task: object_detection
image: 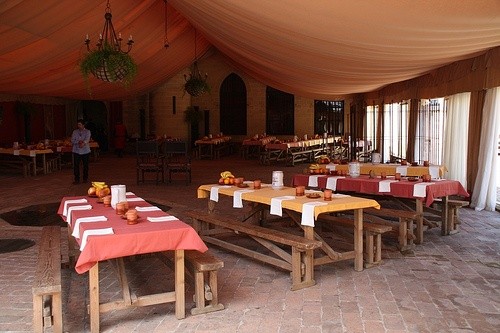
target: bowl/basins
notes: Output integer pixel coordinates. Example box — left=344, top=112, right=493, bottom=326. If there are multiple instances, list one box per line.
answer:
left=411, top=161, right=418, bottom=166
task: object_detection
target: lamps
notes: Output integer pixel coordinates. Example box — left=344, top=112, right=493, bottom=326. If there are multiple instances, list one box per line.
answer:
left=182, top=32, right=211, bottom=98
left=80, top=0, right=137, bottom=97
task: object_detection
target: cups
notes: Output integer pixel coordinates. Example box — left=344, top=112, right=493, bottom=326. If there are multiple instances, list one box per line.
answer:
left=395, top=173, right=401, bottom=181
left=421, top=174, right=431, bottom=182
left=381, top=171, right=386, bottom=180
left=401, top=158, right=407, bottom=164
left=254, top=180, right=261, bottom=189
left=296, top=185, right=305, bottom=196
left=324, top=190, right=332, bottom=200
left=369, top=170, right=377, bottom=178
left=103, top=195, right=111, bottom=207
left=126, top=209, right=138, bottom=224
left=116, top=201, right=129, bottom=215
left=424, top=160, right=429, bottom=166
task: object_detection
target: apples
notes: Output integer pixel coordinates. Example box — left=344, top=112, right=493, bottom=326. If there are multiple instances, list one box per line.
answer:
left=87, top=187, right=110, bottom=197
left=310, top=169, right=319, bottom=173
left=219, top=178, right=234, bottom=184
left=320, top=159, right=328, bottom=164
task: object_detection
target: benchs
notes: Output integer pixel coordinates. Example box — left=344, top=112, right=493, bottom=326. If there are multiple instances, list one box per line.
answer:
left=56, top=192, right=208, bottom=333
left=186, top=193, right=469, bottom=333
left=31, top=223, right=63, bottom=333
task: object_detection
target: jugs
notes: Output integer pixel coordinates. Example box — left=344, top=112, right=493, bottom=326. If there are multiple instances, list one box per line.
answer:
left=372, top=153, right=380, bottom=165
left=272, top=171, right=284, bottom=189
left=349, top=162, right=361, bottom=178
left=110, top=184, right=126, bottom=209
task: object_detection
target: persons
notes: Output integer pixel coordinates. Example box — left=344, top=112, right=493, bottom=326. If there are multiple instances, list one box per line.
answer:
left=72, top=120, right=91, bottom=185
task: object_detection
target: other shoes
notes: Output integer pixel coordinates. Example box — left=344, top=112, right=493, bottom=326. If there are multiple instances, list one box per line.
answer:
left=72, top=181, right=80, bottom=184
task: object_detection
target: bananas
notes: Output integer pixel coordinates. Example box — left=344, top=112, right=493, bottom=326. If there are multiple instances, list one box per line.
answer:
left=320, top=156, right=327, bottom=159
left=221, top=171, right=235, bottom=178
left=91, top=181, right=108, bottom=189
left=310, top=164, right=319, bottom=169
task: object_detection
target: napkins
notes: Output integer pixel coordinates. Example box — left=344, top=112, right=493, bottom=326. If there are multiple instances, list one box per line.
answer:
left=62, top=163, right=438, bottom=257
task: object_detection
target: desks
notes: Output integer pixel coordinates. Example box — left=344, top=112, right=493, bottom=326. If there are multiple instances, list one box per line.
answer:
left=197, top=181, right=381, bottom=281
left=290, top=174, right=470, bottom=244
left=304, top=163, right=429, bottom=177
left=194, top=136, right=342, bottom=166
left=0, top=143, right=98, bottom=176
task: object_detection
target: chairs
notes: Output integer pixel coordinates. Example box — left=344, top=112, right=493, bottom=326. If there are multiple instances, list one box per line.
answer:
left=136, top=141, right=192, bottom=186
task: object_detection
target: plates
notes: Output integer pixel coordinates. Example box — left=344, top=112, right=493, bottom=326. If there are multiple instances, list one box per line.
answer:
left=307, top=193, right=321, bottom=199
left=408, top=177, right=419, bottom=181
